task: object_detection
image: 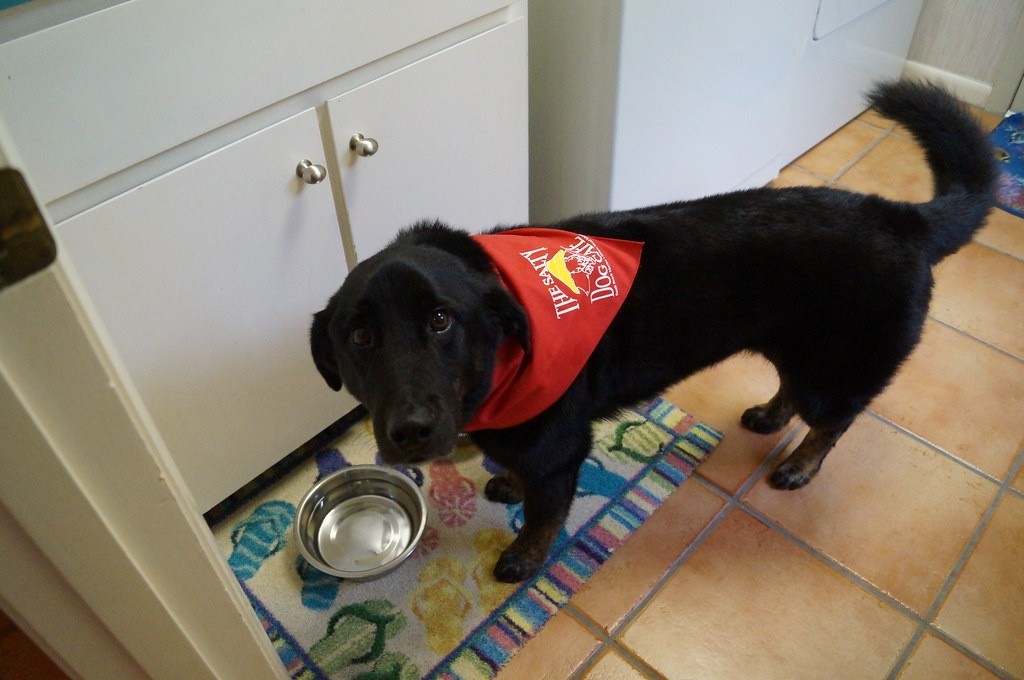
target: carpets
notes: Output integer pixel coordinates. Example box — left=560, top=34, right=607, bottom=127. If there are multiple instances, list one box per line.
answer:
left=203, top=396, right=727, bottom=680
left=985, top=112, right=1024, bottom=221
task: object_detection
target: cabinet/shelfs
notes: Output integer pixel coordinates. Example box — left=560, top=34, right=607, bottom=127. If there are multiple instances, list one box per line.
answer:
left=53, top=11, right=530, bottom=514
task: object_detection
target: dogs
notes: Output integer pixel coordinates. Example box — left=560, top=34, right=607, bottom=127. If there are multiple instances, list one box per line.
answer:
left=307, top=72, right=1003, bottom=587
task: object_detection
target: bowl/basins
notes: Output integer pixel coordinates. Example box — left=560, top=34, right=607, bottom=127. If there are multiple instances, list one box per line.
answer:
left=292, top=462, right=428, bottom=579
